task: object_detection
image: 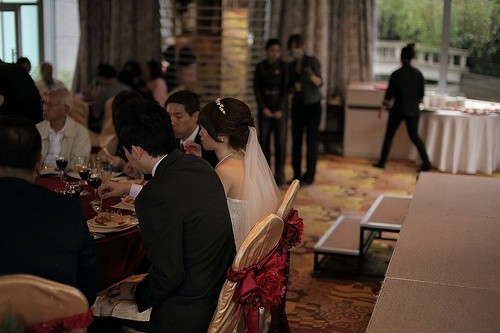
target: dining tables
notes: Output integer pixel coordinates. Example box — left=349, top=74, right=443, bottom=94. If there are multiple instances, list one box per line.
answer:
left=36, top=174, right=141, bottom=290
left=408, top=96, right=499, bottom=175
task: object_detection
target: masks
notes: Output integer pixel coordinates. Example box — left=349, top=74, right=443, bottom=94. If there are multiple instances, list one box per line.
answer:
left=290, top=48, right=304, bottom=59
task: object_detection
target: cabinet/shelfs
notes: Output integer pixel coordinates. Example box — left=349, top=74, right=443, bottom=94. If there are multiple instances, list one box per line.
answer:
left=344, top=83, right=410, bottom=160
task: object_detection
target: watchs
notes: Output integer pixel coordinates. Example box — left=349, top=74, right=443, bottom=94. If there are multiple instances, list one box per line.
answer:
left=130, top=283, right=136, bottom=299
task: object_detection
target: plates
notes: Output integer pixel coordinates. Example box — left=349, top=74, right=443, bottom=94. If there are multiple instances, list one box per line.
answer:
left=110, top=199, right=136, bottom=212
left=86, top=218, right=139, bottom=232
left=92, top=215, right=131, bottom=228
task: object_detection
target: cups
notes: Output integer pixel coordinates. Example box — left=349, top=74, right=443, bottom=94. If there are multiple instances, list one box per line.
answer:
left=74, top=156, right=85, bottom=174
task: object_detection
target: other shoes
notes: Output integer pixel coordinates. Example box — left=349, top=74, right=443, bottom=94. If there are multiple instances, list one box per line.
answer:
left=417, top=160, right=431, bottom=172
left=372, top=160, right=385, bottom=168
left=287, top=172, right=301, bottom=185
left=300, top=172, right=315, bottom=185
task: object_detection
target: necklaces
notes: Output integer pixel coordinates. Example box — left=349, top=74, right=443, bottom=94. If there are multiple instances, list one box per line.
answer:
left=214, top=153, right=233, bottom=170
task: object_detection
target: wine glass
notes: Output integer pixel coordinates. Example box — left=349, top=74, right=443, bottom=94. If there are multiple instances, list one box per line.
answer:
left=79, top=164, right=91, bottom=195
left=98, top=164, right=111, bottom=185
left=87, top=168, right=102, bottom=204
left=55, top=154, right=68, bottom=182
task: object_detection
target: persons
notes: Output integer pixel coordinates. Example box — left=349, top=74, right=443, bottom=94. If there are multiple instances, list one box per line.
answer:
left=0, top=34, right=324, bottom=333
left=373, top=43, right=433, bottom=172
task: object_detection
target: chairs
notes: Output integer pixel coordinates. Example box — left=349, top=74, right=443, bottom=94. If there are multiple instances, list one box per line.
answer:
left=123, top=179, right=301, bottom=333
left=0, top=275, right=90, bottom=333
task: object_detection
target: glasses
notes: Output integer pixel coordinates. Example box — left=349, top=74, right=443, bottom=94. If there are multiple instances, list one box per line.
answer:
left=40, top=98, right=61, bottom=109
